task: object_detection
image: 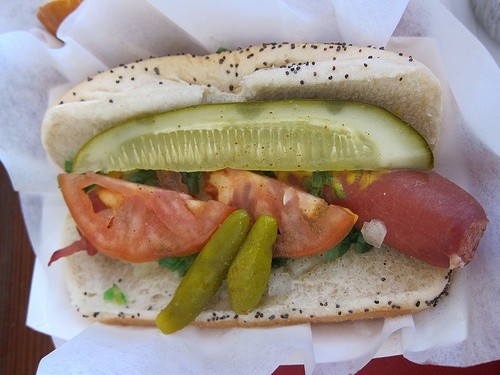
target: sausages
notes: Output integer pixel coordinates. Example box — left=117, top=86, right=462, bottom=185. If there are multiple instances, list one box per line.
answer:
left=272, top=170, right=490, bottom=271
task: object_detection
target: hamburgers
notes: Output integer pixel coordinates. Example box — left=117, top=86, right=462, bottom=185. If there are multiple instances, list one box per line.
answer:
left=41, top=44, right=456, bottom=328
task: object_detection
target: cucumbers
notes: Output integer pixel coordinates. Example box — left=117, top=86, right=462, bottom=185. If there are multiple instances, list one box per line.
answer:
left=73, top=98, right=435, bottom=174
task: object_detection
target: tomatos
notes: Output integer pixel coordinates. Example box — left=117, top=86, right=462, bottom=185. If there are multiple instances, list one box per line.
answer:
left=55, top=171, right=356, bottom=262
